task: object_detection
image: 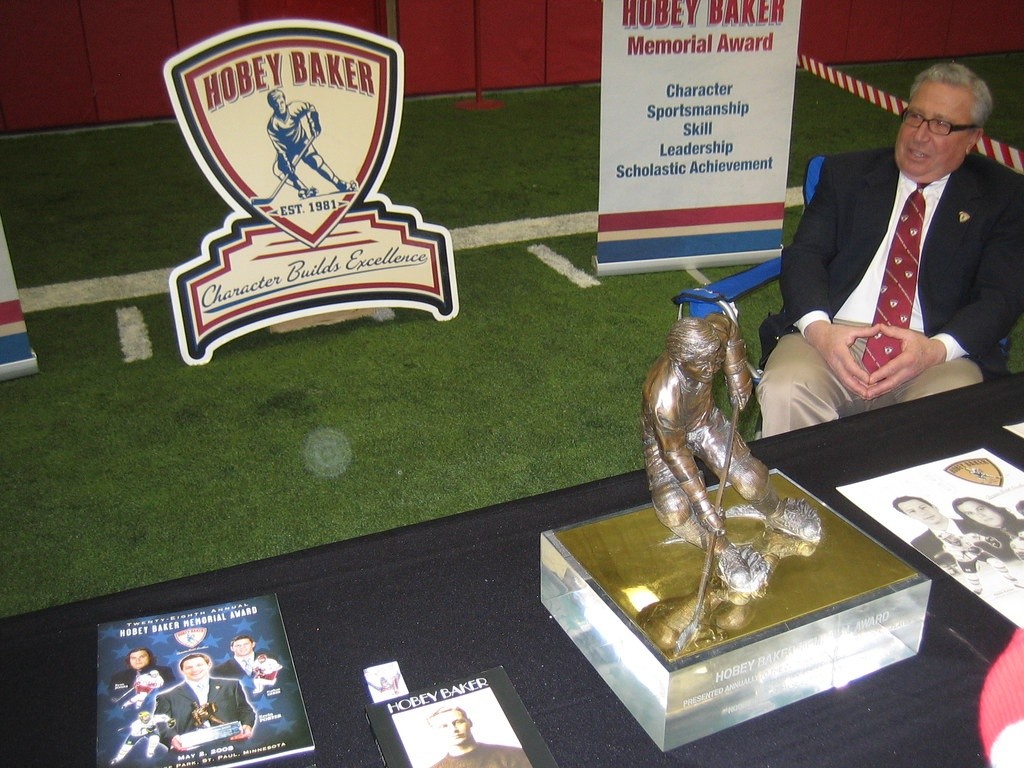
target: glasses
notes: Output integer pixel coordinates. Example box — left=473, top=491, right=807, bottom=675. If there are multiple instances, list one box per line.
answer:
left=901, top=108, right=978, bottom=136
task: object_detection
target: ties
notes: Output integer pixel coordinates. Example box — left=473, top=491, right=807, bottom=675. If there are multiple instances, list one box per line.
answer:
left=861, top=182, right=930, bottom=382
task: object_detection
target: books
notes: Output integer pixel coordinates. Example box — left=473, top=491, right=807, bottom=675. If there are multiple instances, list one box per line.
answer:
left=95, top=592, right=315, bottom=768
left=364, top=663, right=562, bottom=768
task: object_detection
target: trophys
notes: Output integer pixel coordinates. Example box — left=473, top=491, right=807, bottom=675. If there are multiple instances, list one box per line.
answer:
left=541, top=312, right=932, bottom=753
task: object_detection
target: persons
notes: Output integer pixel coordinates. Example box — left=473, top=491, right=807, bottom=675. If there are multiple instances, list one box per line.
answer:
left=253, top=654, right=283, bottom=695
left=152, top=654, right=257, bottom=753
left=938, top=531, right=1024, bottom=594
left=952, top=498, right=1024, bottom=560
left=110, top=711, right=176, bottom=764
left=427, top=705, right=513, bottom=768
left=121, top=670, right=164, bottom=709
left=892, top=496, right=965, bottom=566
left=209, top=634, right=279, bottom=679
left=109, top=647, right=176, bottom=693
left=756, top=62, right=1024, bottom=438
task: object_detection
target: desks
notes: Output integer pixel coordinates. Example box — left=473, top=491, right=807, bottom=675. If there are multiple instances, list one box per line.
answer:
left=0, top=371, right=1024, bottom=768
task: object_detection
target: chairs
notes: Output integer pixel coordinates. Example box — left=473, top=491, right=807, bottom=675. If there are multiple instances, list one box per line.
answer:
left=671, top=154, right=1011, bottom=441
